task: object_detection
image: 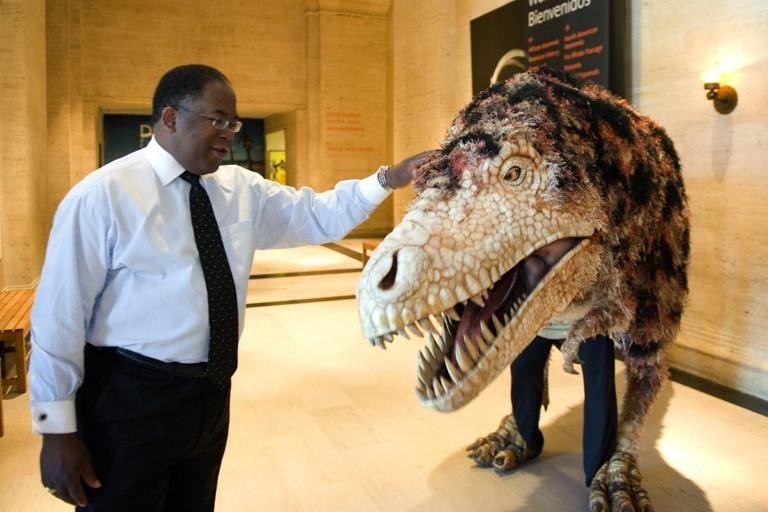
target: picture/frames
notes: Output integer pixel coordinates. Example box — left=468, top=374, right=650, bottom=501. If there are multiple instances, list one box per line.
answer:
left=269, top=150, right=287, bottom=186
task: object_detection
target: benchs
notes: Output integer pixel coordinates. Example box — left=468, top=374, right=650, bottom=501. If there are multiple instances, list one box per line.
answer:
left=0, top=289, right=40, bottom=393
left=362, top=240, right=385, bottom=271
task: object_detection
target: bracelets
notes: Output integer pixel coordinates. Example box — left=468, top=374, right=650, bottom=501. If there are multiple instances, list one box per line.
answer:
left=378, top=169, right=396, bottom=193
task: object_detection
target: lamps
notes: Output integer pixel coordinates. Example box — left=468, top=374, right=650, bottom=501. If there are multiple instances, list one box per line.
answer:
left=700, top=70, right=738, bottom=114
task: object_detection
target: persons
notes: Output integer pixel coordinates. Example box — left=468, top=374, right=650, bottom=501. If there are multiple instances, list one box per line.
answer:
left=29, top=64, right=445, bottom=512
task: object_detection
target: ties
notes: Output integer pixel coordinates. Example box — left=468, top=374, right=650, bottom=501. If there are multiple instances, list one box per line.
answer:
left=180, top=169, right=239, bottom=391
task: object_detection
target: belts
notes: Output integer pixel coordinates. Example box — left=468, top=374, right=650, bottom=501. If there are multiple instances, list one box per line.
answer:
left=87, top=346, right=235, bottom=382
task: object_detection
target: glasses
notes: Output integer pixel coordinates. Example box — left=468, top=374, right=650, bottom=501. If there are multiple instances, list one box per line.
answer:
left=174, top=101, right=242, bottom=134
